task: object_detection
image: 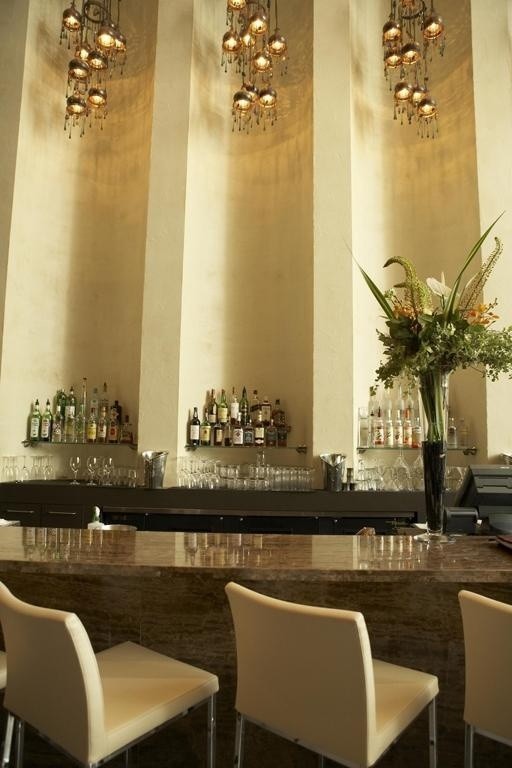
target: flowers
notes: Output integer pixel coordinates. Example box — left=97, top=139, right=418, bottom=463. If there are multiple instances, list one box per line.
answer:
left=341, top=211, right=512, bottom=442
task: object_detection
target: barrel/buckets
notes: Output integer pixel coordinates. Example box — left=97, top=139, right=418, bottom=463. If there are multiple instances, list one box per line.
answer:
left=498, top=454, right=512, bottom=466
left=143, top=451, right=168, bottom=489
left=320, top=453, right=346, bottom=491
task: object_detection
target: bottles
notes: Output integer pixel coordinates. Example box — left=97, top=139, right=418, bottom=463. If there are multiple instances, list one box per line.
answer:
left=359, top=383, right=469, bottom=450
left=183, top=531, right=272, bottom=567
left=366, top=535, right=423, bottom=570
left=22, top=527, right=134, bottom=562
left=28, top=377, right=133, bottom=444
left=190, top=385, right=291, bottom=447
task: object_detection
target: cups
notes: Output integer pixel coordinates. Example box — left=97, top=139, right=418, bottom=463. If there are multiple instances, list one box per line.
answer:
left=221, top=451, right=314, bottom=492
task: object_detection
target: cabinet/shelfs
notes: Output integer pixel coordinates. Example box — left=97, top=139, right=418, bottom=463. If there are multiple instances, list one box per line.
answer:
left=0, top=503, right=82, bottom=529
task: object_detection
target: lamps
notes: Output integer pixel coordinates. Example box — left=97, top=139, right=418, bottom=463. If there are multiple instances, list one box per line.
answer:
left=383, top=0, right=447, bottom=139
left=58, top=0, right=126, bottom=140
left=220, top=0, right=290, bottom=134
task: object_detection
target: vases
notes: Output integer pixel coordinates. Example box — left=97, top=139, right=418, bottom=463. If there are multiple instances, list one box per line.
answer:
left=423, top=442, right=447, bottom=541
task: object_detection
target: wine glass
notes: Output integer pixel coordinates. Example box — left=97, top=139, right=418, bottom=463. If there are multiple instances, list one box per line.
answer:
left=179, top=450, right=220, bottom=491
left=358, top=450, right=466, bottom=492
left=1, top=456, right=137, bottom=489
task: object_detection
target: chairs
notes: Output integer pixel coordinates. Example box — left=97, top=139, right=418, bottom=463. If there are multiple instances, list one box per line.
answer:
left=458, top=587, right=511, bottom=768
left=226, top=582, right=441, bottom=766
left=0, top=582, right=220, bottom=768
